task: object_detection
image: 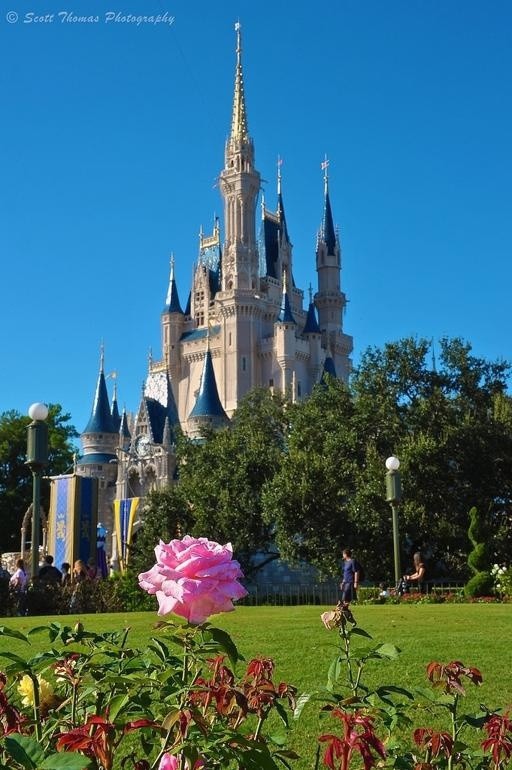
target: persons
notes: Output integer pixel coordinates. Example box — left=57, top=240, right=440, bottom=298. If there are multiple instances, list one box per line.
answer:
left=0, top=554, right=104, bottom=618
left=370, top=580, right=391, bottom=602
left=338, top=549, right=360, bottom=605
left=403, top=551, right=433, bottom=594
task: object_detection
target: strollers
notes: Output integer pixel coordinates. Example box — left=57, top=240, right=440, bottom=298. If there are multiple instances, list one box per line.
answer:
left=367, top=578, right=411, bottom=606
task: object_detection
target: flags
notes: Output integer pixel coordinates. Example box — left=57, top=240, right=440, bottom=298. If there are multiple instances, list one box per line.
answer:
left=114, top=496, right=140, bottom=575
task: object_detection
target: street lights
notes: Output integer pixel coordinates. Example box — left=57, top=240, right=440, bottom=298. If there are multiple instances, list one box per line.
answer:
left=21, top=402, right=50, bottom=616
left=380, top=455, right=409, bottom=603
